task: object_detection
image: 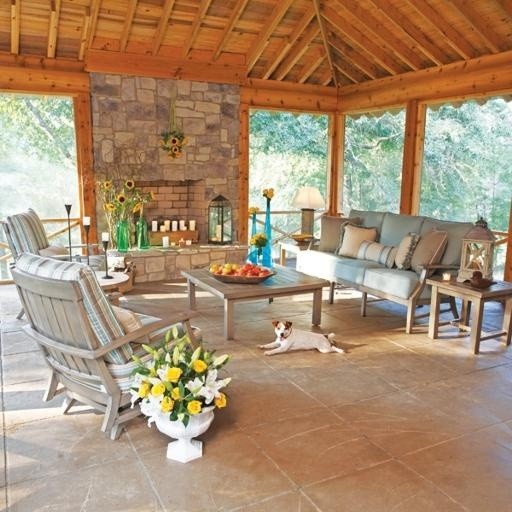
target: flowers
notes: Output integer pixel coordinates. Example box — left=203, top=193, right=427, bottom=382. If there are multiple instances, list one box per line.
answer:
left=291, top=234, right=313, bottom=241
left=133, top=189, right=154, bottom=216
left=134, top=325, right=230, bottom=424
left=159, top=83, right=190, bottom=160
left=98, top=179, right=134, bottom=218
left=248, top=188, right=276, bottom=264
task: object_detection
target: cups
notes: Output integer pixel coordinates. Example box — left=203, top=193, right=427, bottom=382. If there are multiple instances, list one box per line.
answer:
left=151, top=220, right=196, bottom=248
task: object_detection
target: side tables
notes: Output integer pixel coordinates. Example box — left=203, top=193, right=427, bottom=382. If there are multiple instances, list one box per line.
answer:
left=93, top=271, right=129, bottom=307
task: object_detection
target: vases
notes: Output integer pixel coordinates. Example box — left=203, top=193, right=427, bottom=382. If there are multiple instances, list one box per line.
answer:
left=136, top=215, right=150, bottom=249
left=156, top=405, right=215, bottom=464
left=115, top=219, right=129, bottom=251
left=295, top=242, right=310, bottom=249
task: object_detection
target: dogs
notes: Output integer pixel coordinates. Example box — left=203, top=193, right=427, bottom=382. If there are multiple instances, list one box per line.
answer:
left=256, top=320, right=346, bottom=356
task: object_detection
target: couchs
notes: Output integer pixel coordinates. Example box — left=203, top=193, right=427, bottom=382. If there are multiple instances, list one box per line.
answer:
left=296, top=209, right=474, bottom=332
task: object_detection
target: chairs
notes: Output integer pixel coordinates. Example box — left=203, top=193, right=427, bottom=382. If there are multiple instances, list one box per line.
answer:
left=10, top=253, right=203, bottom=440
left=0, top=207, right=105, bottom=319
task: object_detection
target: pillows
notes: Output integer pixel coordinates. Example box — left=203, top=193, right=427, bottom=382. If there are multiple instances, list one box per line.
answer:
left=39, top=240, right=69, bottom=257
left=320, top=215, right=448, bottom=275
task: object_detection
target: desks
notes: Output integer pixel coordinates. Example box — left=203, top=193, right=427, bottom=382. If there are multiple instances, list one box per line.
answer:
left=425, top=274, right=512, bottom=354
left=278, top=239, right=320, bottom=268
left=181, top=262, right=331, bottom=341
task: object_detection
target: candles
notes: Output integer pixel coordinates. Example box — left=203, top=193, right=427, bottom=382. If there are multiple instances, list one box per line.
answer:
left=83, top=217, right=90, bottom=225
left=102, top=232, right=110, bottom=241
left=65, top=197, right=72, bottom=205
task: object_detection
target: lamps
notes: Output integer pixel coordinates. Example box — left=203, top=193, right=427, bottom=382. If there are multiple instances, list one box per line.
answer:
left=293, top=187, right=326, bottom=242
